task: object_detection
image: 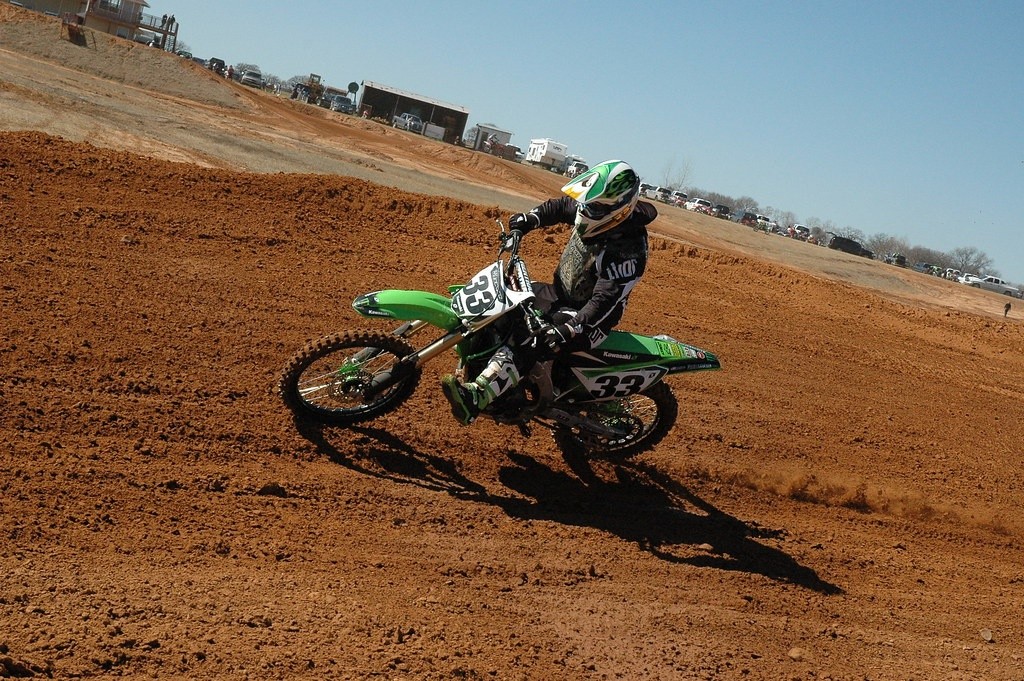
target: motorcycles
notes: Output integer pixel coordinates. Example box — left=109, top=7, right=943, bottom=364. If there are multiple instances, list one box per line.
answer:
left=483, top=140, right=505, bottom=156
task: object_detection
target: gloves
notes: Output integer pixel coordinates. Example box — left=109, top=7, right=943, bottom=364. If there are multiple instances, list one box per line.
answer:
left=509, top=212, right=538, bottom=235
left=530, top=324, right=571, bottom=352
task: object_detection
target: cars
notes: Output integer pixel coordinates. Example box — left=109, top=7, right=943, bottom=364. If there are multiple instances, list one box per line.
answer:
left=278, top=216, right=720, bottom=460
left=242, top=70, right=264, bottom=89
left=641, top=182, right=811, bottom=240
left=330, top=96, right=354, bottom=115
left=176, top=49, right=226, bottom=76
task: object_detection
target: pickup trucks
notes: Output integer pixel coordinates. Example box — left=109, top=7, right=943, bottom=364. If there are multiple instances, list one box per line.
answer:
left=391, top=113, right=423, bottom=134
left=964, top=274, right=1019, bottom=297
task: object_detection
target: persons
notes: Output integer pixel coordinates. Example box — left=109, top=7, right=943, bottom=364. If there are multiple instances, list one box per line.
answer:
left=441, top=160, right=658, bottom=426
left=926, top=266, right=957, bottom=282
left=167, top=14, right=175, bottom=34
left=1003, top=301, right=1011, bottom=318
left=158, top=13, right=167, bottom=30
left=226, top=65, right=234, bottom=81
left=674, top=198, right=797, bottom=240
left=488, top=133, right=499, bottom=150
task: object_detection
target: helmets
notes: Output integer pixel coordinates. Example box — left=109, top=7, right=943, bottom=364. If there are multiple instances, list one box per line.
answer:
left=560, top=160, right=640, bottom=238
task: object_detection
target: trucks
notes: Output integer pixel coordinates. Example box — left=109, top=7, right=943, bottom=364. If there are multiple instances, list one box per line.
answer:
left=526, top=139, right=567, bottom=169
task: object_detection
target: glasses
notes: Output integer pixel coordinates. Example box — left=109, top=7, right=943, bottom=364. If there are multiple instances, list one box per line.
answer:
left=577, top=202, right=611, bottom=217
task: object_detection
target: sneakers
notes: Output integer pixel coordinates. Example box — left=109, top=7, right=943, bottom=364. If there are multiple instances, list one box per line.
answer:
left=442, top=374, right=489, bottom=426
